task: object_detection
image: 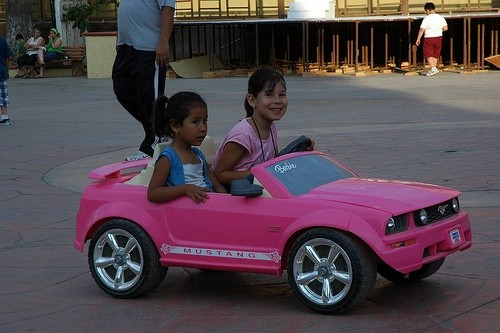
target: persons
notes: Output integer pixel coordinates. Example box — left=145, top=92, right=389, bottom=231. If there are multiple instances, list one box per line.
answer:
left=17, top=28, right=45, bottom=79
left=0, top=36, right=12, bottom=126
left=15, top=35, right=27, bottom=78
left=210, top=67, right=314, bottom=191
left=112, top=0, right=175, bottom=162
left=148, top=91, right=229, bottom=204
left=415, top=2, right=449, bottom=77
left=33, top=28, right=63, bottom=79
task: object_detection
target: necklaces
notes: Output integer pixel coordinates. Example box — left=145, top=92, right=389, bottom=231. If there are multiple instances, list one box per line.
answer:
left=251, top=116, right=277, bottom=162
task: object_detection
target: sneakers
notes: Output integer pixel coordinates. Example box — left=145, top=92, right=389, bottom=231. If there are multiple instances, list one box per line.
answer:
left=427, top=68, right=440, bottom=76
left=125, top=150, right=153, bottom=161
left=0, top=119, right=11, bottom=125
left=151, top=135, right=169, bottom=149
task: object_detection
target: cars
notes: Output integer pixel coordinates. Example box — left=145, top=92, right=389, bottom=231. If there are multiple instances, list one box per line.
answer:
left=74, top=150, right=473, bottom=315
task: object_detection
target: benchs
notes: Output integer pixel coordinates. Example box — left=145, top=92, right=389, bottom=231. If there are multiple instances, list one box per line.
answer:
left=126, top=136, right=217, bottom=186
left=48, top=46, right=86, bottom=76
